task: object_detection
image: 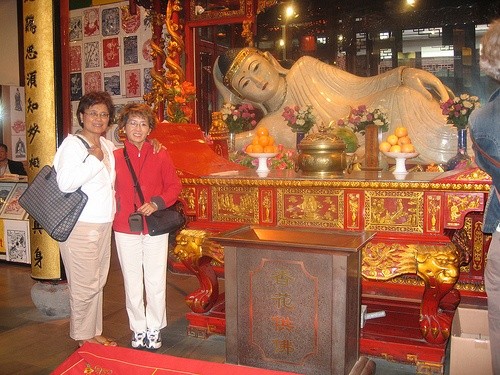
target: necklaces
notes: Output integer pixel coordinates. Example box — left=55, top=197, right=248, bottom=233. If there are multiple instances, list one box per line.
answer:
left=0, top=162, right=8, bottom=177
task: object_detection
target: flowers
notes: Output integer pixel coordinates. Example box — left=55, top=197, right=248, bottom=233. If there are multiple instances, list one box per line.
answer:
left=165, top=80, right=486, bottom=172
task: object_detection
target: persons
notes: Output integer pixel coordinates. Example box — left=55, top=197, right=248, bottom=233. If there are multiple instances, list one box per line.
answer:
left=49, top=92, right=119, bottom=350
left=212, top=47, right=478, bottom=166
left=0, top=143, right=28, bottom=176
left=107, top=104, right=184, bottom=351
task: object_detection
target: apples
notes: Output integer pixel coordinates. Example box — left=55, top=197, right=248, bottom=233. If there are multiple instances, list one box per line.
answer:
left=379, top=127, right=415, bottom=153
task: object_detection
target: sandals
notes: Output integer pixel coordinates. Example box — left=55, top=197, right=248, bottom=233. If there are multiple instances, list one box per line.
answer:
left=94, top=335, right=118, bottom=347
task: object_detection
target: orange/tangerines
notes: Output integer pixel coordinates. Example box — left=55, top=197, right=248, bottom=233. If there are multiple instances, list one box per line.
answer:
left=245, top=127, right=278, bottom=154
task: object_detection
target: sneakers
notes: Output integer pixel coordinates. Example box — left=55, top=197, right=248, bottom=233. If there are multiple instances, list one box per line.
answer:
left=145, top=330, right=162, bottom=349
left=131, top=330, right=146, bottom=349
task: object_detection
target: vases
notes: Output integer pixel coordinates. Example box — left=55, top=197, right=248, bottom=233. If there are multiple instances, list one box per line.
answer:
left=445, top=129, right=474, bottom=171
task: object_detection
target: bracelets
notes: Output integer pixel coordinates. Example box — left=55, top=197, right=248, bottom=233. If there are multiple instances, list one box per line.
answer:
left=152, top=201, right=159, bottom=210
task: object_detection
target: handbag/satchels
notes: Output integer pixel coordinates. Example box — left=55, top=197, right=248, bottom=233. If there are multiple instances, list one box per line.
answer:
left=145, top=205, right=186, bottom=236
left=18, top=166, right=88, bottom=242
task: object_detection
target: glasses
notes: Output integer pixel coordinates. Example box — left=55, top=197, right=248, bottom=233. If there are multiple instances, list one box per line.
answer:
left=125, top=121, right=149, bottom=128
left=83, top=111, right=110, bottom=120
